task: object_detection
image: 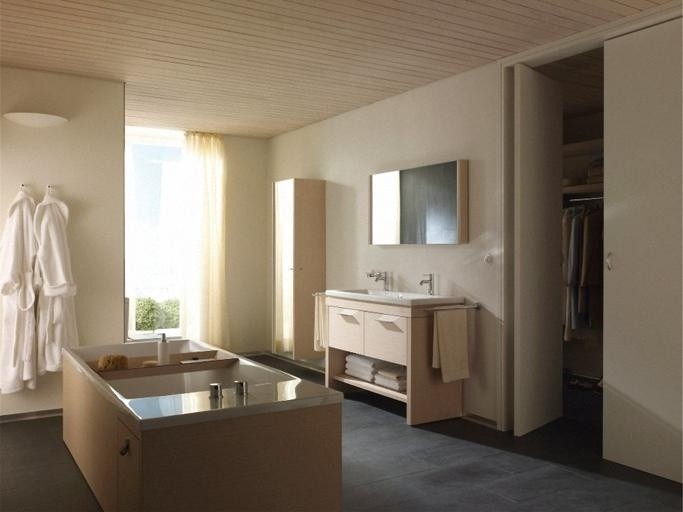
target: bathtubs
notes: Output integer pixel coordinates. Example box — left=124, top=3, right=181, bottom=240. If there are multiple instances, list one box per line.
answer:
left=61, top=335, right=346, bottom=436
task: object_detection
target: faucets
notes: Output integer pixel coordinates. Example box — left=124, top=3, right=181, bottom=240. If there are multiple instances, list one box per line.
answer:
left=375, top=271, right=394, bottom=291
left=420, top=273, right=433, bottom=295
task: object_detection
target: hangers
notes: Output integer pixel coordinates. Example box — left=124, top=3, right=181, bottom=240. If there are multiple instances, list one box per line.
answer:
left=562, top=196, right=603, bottom=221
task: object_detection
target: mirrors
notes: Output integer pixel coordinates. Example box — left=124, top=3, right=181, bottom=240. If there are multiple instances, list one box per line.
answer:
left=366, top=159, right=470, bottom=245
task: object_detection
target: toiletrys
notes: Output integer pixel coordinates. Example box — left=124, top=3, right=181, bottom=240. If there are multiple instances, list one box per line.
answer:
left=156, top=332, right=169, bottom=366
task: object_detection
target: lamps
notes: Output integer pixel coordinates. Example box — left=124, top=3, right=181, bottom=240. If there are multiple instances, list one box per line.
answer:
left=2, top=95, right=73, bottom=132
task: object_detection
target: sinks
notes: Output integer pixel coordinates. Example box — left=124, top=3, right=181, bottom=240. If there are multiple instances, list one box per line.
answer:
left=324, top=286, right=466, bottom=307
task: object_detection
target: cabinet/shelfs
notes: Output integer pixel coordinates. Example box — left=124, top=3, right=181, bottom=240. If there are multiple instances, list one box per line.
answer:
left=496, top=13, right=681, bottom=482
left=323, top=297, right=475, bottom=428
left=59, top=353, right=344, bottom=512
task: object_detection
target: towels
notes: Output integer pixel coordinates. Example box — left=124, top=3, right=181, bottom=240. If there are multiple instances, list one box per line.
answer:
left=310, top=291, right=326, bottom=353
left=431, top=304, right=475, bottom=385
left=342, top=354, right=405, bottom=390
